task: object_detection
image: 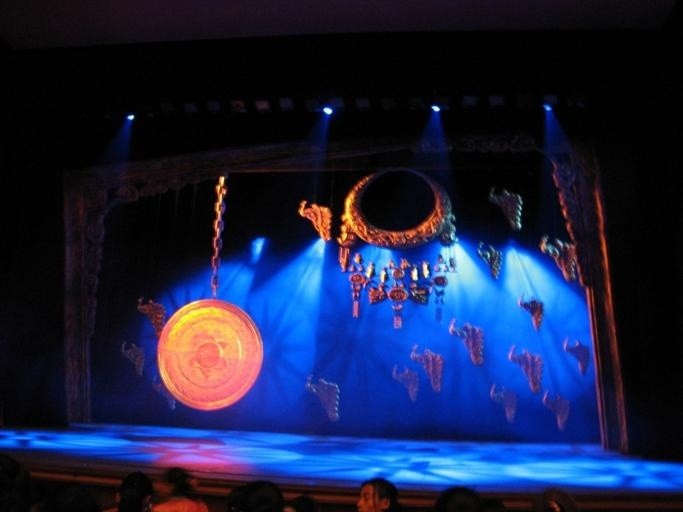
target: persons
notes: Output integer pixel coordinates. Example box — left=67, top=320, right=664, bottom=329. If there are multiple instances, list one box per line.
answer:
left=99, top=469, right=156, bottom=511
left=148, top=464, right=209, bottom=510
left=281, top=495, right=322, bottom=512
left=353, top=477, right=398, bottom=511
